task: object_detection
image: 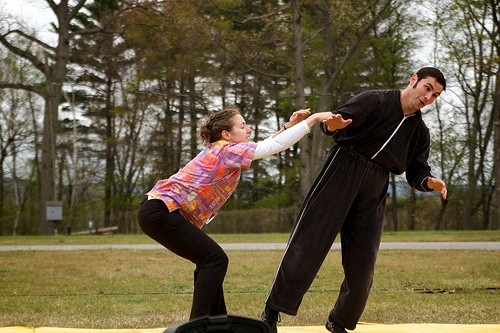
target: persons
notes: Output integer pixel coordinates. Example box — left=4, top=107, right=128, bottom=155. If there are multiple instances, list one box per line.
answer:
left=259, top=67, right=449, bottom=333
left=138, top=109, right=334, bottom=321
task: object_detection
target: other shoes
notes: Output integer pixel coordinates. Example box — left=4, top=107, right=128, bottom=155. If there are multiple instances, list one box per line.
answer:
left=261, top=309, right=279, bottom=333
left=325, top=315, right=348, bottom=333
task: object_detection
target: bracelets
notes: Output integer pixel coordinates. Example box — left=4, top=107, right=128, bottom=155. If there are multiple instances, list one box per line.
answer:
left=283, top=123, right=287, bottom=130
